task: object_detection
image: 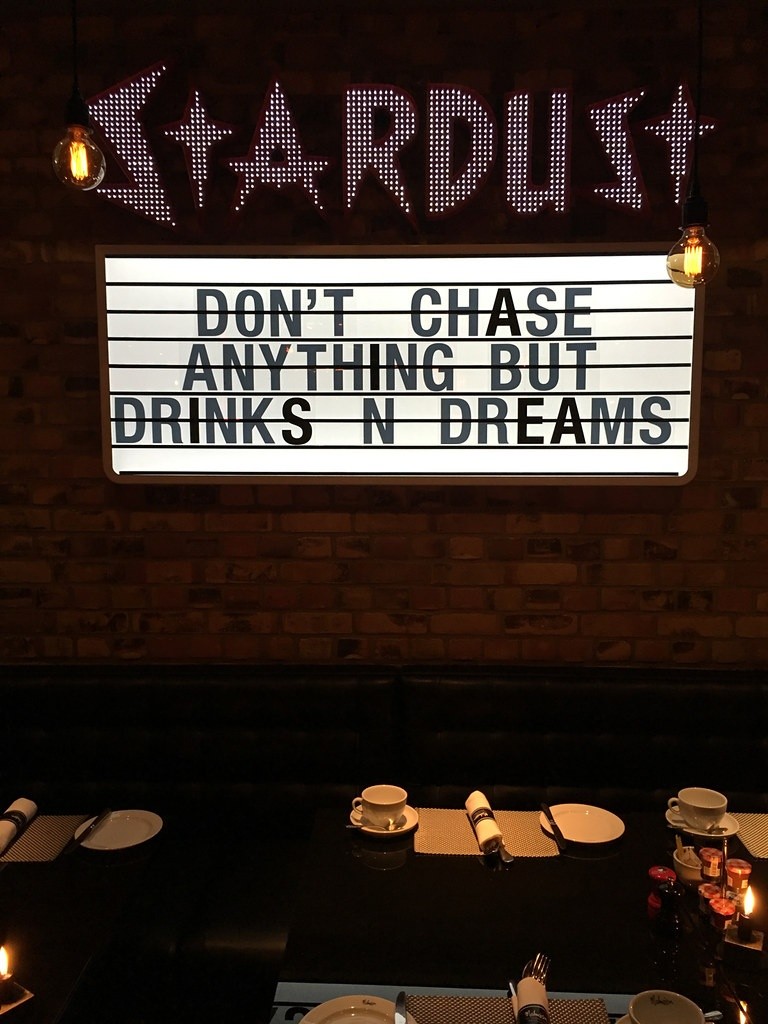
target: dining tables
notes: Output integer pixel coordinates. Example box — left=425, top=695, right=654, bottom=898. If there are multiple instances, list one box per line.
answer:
left=0, top=781, right=768, bottom=1024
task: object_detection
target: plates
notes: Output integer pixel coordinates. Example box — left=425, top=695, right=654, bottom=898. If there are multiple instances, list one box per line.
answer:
left=539, top=804, right=625, bottom=842
left=666, top=806, right=739, bottom=839
left=350, top=804, right=419, bottom=836
left=298, top=995, right=418, bottom=1024
left=75, top=809, right=163, bottom=850
left=615, top=1014, right=714, bottom=1024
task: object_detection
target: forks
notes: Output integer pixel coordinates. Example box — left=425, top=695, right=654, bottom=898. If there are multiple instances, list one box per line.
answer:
left=531, top=953, right=552, bottom=984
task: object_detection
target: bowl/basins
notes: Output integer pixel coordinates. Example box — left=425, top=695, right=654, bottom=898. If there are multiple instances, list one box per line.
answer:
left=673, top=847, right=702, bottom=885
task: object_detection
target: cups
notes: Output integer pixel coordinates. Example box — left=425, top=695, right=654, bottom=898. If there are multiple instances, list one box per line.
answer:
left=668, top=787, right=728, bottom=828
left=628, top=989, right=705, bottom=1024
left=352, top=784, right=408, bottom=827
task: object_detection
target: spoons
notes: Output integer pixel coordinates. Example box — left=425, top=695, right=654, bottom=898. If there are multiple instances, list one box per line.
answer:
left=522, top=960, right=534, bottom=979
left=346, top=822, right=404, bottom=831
left=667, top=825, right=728, bottom=834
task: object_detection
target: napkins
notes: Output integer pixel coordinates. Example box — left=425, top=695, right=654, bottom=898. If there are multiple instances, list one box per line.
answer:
left=465, top=790, right=502, bottom=854
left=0, top=798, right=37, bottom=856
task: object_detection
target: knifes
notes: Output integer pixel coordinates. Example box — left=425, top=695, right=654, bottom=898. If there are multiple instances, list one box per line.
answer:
left=394, top=991, right=407, bottom=1024
left=541, top=803, right=566, bottom=850
left=59, top=807, right=111, bottom=854
